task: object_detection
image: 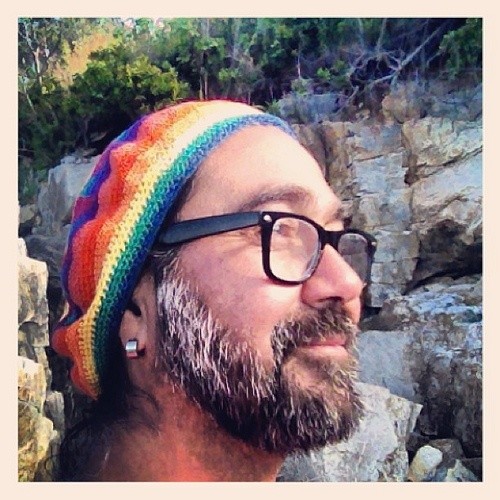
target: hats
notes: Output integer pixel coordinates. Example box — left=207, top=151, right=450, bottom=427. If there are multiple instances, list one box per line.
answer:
left=49, top=100, right=297, bottom=404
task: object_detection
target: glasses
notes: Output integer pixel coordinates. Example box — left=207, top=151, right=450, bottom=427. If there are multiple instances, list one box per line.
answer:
left=155, top=210, right=378, bottom=285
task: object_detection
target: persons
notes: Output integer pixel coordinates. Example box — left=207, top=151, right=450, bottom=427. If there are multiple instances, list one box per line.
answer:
left=29, top=100, right=379, bottom=483
